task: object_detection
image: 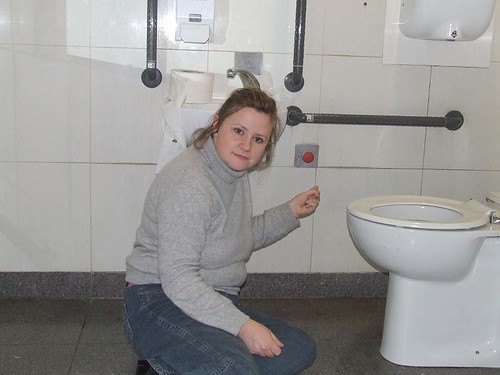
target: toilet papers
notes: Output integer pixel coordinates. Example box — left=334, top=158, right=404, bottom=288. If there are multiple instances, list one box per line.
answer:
left=152, top=68, right=214, bottom=176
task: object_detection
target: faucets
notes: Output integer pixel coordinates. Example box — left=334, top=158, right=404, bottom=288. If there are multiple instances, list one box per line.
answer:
left=227, top=68, right=260, bottom=91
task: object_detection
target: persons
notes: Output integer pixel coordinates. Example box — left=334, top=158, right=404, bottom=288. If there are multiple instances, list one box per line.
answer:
left=121, top=88, right=322, bottom=375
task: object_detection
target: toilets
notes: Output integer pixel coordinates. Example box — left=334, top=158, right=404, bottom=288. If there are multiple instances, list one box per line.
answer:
left=344, top=191, right=499, bottom=368
